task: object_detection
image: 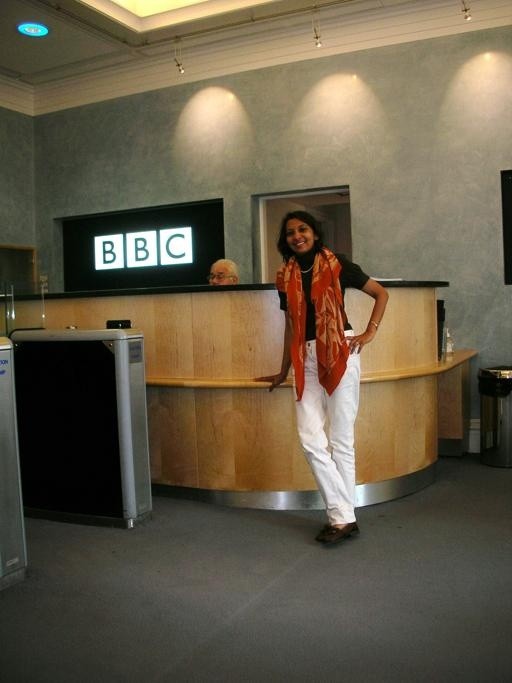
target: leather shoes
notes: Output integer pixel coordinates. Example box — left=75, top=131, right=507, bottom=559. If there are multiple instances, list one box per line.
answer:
left=315, top=520, right=361, bottom=546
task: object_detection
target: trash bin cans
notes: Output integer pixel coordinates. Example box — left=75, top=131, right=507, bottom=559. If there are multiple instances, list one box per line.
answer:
left=478, top=366, right=512, bottom=468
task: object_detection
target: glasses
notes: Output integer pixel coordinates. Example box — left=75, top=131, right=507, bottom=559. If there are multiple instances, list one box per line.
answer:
left=206, top=273, right=236, bottom=281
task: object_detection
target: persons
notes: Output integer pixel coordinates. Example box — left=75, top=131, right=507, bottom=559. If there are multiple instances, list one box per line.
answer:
left=208, top=256, right=239, bottom=286
left=252, top=206, right=390, bottom=547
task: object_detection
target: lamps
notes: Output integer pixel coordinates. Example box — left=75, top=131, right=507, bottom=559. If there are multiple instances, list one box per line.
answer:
left=171, top=0, right=472, bottom=75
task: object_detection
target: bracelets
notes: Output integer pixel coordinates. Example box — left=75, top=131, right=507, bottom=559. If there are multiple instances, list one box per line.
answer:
left=368, top=316, right=379, bottom=332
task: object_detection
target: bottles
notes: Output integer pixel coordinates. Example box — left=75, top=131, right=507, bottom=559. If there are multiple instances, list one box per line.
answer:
left=444, top=323, right=455, bottom=358
